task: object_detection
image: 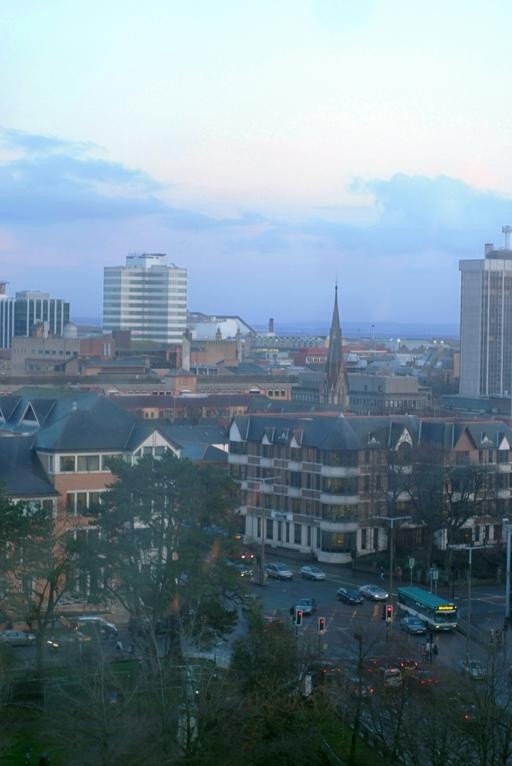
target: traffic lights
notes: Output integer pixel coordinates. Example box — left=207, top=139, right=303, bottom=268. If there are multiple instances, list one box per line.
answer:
left=296, top=609, right=303, bottom=626
left=385, top=605, right=393, bottom=623
left=319, top=616, right=326, bottom=634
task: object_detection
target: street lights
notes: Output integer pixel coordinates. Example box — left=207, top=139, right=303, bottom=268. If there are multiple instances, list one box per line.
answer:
left=374, top=515, right=413, bottom=595
left=448, top=545, right=494, bottom=641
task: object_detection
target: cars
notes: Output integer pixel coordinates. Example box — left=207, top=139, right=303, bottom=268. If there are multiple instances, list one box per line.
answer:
left=3, top=629, right=38, bottom=647
left=78, top=617, right=119, bottom=639
left=296, top=596, right=317, bottom=615
left=242, top=593, right=284, bottom=629
left=309, top=657, right=440, bottom=699
left=337, top=582, right=391, bottom=606
left=399, top=616, right=427, bottom=635
left=233, top=546, right=328, bottom=582
left=459, top=659, right=487, bottom=680
left=450, top=703, right=484, bottom=729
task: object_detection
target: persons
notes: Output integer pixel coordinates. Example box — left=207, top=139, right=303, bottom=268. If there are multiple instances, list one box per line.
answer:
left=379, top=566, right=385, bottom=584
left=395, top=567, right=401, bottom=584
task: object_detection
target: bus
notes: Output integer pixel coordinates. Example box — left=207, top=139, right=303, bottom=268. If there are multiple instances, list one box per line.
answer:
left=398, top=585, right=457, bottom=633
